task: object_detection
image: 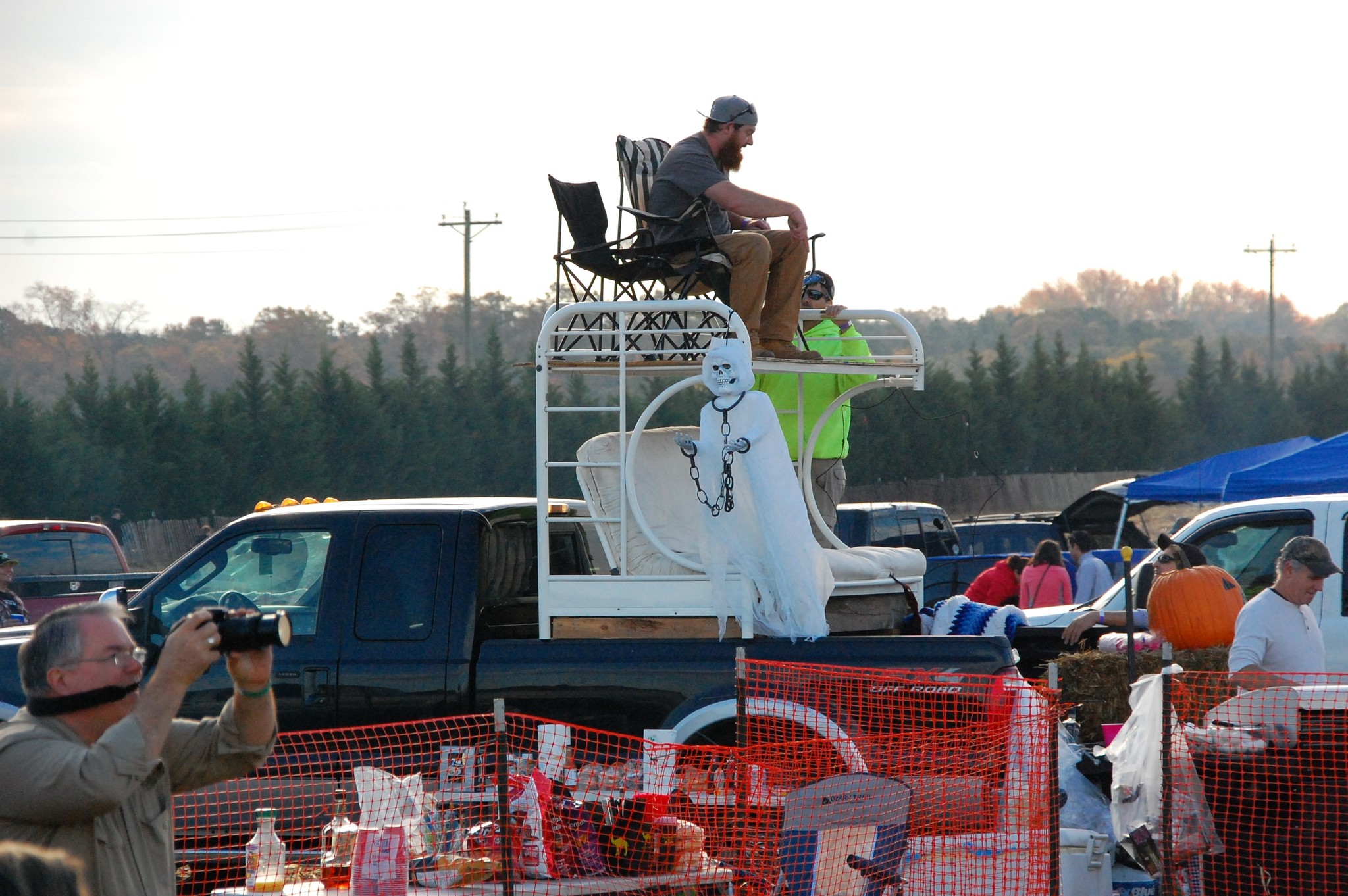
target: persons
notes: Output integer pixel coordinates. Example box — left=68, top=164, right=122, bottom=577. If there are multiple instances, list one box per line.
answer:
left=641, top=95, right=826, bottom=362
left=86, top=508, right=128, bottom=547
left=0, top=601, right=278, bottom=896
left=192, top=525, right=211, bottom=546
left=0, top=551, right=30, bottom=628
left=1228, top=535, right=1344, bottom=696
left=1062, top=533, right=1207, bottom=676
left=0, top=840, right=87, bottom=896
left=672, top=338, right=836, bottom=642
left=962, top=531, right=1118, bottom=622
left=733, top=270, right=876, bottom=548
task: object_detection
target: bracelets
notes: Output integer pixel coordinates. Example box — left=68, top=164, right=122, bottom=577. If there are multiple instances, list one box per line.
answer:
left=740, top=219, right=750, bottom=230
left=233, top=681, right=273, bottom=698
left=838, top=320, right=852, bottom=329
left=1098, top=611, right=1105, bottom=624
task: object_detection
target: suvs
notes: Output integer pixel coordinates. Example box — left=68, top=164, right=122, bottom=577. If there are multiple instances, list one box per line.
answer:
left=1018, top=491, right=1348, bottom=674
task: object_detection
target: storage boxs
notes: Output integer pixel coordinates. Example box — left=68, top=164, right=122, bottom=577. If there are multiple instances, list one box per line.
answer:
left=643, top=728, right=676, bottom=795
left=1112, top=862, right=1160, bottom=896
left=899, top=828, right=1112, bottom=896
left=537, top=724, right=571, bottom=781
left=439, top=745, right=486, bottom=793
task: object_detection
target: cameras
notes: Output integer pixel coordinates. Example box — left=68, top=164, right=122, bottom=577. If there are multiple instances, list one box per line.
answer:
left=187, top=606, right=294, bottom=654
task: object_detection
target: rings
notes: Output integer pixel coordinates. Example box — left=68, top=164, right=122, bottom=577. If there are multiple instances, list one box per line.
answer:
left=205, top=636, right=218, bottom=648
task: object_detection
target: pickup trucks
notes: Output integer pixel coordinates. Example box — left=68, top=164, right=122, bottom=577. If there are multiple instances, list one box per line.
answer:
left=0, top=519, right=165, bottom=625
left=831, top=500, right=1159, bottom=557
left=1, top=494, right=1024, bottom=843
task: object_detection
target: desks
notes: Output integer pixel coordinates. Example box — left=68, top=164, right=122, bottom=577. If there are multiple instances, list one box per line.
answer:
left=211, top=868, right=733, bottom=896
left=431, top=787, right=787, bottom=821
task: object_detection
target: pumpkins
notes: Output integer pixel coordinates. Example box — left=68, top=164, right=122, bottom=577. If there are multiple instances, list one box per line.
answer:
left=1146, top=546, right=1247, bottom=652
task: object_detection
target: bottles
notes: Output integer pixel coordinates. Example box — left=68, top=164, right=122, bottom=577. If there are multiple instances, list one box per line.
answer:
left=522, top=753, right=534, bottom=776
left=244, top=807, right=287, bottom=893
left=562, top=745, right=577, bottom=791
left=707, top=759, right=716, bottom=794
left=320, top=790, right=358, bottom=888
left=1061, top=708, right=1081, bottom=745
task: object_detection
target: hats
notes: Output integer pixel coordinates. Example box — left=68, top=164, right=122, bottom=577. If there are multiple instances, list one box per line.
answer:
left=1158, top=533, right=1206, bottom=571
left=0, top=552, right=18, bottom=568
left=802, top=270, right=835, bottom=298
left=1280, top=535, right=1345, bottom=577
left=697, top=96, right=758, bottom=126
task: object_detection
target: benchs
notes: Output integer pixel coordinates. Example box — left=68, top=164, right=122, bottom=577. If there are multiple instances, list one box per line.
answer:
left=576, top=424, right=928, bottom=588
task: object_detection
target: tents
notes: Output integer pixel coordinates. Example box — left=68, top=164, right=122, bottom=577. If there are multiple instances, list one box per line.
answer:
left=1114, top=430, right=1348, bottom=549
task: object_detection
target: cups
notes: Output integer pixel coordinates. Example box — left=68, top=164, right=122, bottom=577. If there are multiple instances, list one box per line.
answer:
left=350, top=824, right=409, bottom=896
left=1100, top=723, right=1123, bottom=747
left=10, top=614, right=24, bottom=624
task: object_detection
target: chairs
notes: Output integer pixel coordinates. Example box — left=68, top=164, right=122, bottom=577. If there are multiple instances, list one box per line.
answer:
left=549, top=135, right=825, bottom=362
left=696, top=772, right=914, bottom=896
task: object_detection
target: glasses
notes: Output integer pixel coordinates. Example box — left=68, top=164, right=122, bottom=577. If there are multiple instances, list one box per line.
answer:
left=805, top=290, right=827, bottom=300
left=727, top=103, right=756, bottom=125
left=1157, top=555, right=1175, bottom=565
left=57, top=647, right=147, bottom=667
